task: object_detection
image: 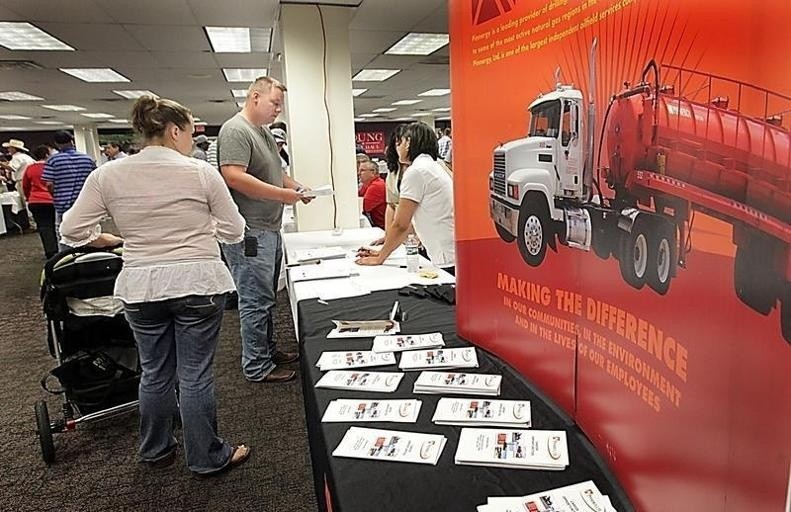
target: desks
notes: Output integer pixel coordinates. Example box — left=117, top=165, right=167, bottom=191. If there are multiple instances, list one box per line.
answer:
left=281, top=227, right=635, bottom=512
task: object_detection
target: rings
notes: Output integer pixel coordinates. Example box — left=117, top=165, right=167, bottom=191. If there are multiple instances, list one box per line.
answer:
left=400, top=265, right=422, bottom=269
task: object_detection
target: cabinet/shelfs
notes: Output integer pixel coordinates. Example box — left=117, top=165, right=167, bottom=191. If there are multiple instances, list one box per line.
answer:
left=404, top=233, right=420, bottom=274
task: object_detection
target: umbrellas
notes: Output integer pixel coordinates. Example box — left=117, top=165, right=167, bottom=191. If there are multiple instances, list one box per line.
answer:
left=272, top=351, right=299, bottom=363
left=263, top=367, right=295, bottom=382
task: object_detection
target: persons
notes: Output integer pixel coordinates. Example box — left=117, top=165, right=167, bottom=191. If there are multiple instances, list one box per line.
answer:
left=0, top=139, right=142, bottom=259
left=216, top=76, right=316, bottom=383
left=59, top=94, right=251, bottom=473
left=355, top=121, right=455, bottom=276
left=191, top=135, right=220, bottom=172
left=269, top=122, right=289, bottom=173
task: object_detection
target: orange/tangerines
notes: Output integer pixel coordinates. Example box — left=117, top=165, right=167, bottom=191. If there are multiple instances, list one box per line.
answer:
left=29, top=247, right=179, bottom=465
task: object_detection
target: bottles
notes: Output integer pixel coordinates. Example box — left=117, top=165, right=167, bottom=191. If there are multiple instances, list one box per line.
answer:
left=269, top=128, right=287, bottom=145
left=194, top=135, right=208, bottom=145
left=2, top=139, right=30, bottom=152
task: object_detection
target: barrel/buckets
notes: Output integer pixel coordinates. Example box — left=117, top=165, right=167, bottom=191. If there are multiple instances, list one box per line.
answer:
left=225, top=444, right=250, bottom=473
